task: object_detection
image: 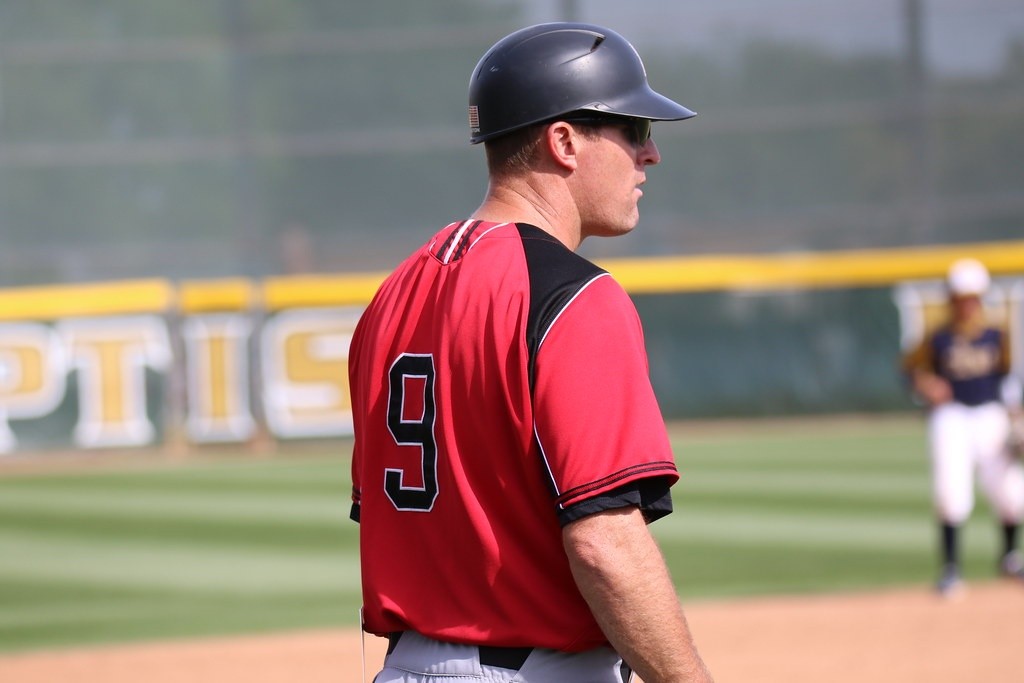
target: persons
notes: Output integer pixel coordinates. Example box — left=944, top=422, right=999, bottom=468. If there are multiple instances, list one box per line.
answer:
left=345, top=20, right=717, bottom=683
left=902, top=257, right=1024, bottom=603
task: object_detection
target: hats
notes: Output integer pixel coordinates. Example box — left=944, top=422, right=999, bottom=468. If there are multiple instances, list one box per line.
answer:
left=946, top=259, right=989, bottom=302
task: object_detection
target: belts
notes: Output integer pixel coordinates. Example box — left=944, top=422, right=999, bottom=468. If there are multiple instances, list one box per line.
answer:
left=386, top=632, right=532, bottom=671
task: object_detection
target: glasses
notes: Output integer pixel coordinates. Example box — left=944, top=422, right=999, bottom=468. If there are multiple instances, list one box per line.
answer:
left=558, top=116, right=651, bottom=146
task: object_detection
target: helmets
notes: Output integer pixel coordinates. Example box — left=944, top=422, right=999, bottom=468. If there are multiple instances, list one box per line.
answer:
left=469, top=22, right=698, bottom=144
